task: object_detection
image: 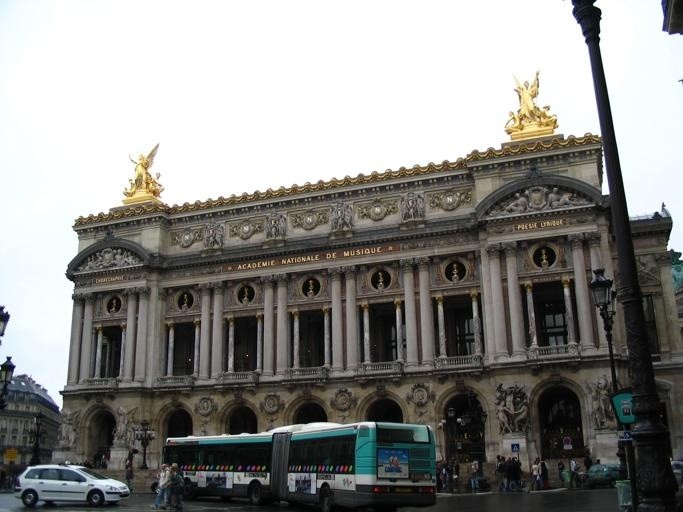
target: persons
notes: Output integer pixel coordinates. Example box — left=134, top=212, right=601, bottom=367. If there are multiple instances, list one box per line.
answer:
left=126, top=417, right=138, bottom=442
left=635, top=255, right=661, bottom=285
left=513, top=403, right=527, bottom=432
left=263, top=211, right=285, bottom=238
left=125, top=458, right=134, bottom=492
left=100, top=453, right=107, bottom=472
left=203, top=224, right=224, bottom=248
left=541, top=188, right=589, bottom=209
left=585, top=373, right=618, bottom=432
left=496, top=400, right=514, bottom=433
left=65, top=459, right=100, bottom=468
left=149, top=172, right=164, bottom=196
left=134, top=154, right=149, bottom=190
left=151, top=461, right=186, bottom=511
left=540, top=104, right=559, bottom=129
left=514, top=69, right=540, bottom=119
left=504, top=111, right=522, bottom=134
left=122, top=179, right=135, bottom=196
left=329, top=203, right=353, bottom=231
left=434, top=444, right=601, bottom=494
left=502, top=383, right=525, bottom=411
left=398, top=193, right=426, bottom=220
left=77, top=247, right=139, bottom=271
left=505, top=192, right=533, bottom=212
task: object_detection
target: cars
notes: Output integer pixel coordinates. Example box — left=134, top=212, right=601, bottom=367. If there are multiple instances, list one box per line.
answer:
left=671, top=461, right=682, bottom=484
left=13, top=460, right=129, bottom=507
left=588, top=464, right=618, bottom=487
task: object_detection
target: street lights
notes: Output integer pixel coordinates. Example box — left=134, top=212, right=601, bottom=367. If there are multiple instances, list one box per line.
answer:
left=590, top=269, right=628, bottom=480
left=134, top=420, right=156, bottom=469
left=29, top=409, right=48, bottom=464
left=436, top=407, right=466, bottom=493
left=0, top=305, right=15, bottom=408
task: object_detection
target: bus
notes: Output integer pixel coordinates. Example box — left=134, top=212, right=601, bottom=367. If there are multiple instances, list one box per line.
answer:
left=164, top=420, right=437, bottom=511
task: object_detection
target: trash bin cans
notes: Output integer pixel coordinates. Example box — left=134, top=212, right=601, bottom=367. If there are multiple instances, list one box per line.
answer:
left=561, top=470, right=571, bottom=489
left=616, top=480, right=633, bottom=512
